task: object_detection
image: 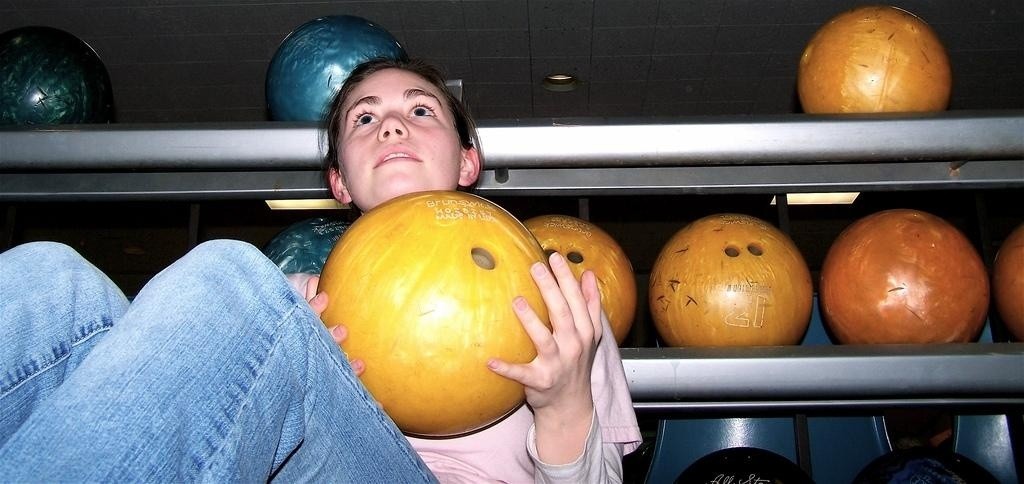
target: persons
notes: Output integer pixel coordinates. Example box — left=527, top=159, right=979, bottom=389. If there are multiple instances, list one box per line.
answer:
left=0, top=53, right=644, bottom=484
